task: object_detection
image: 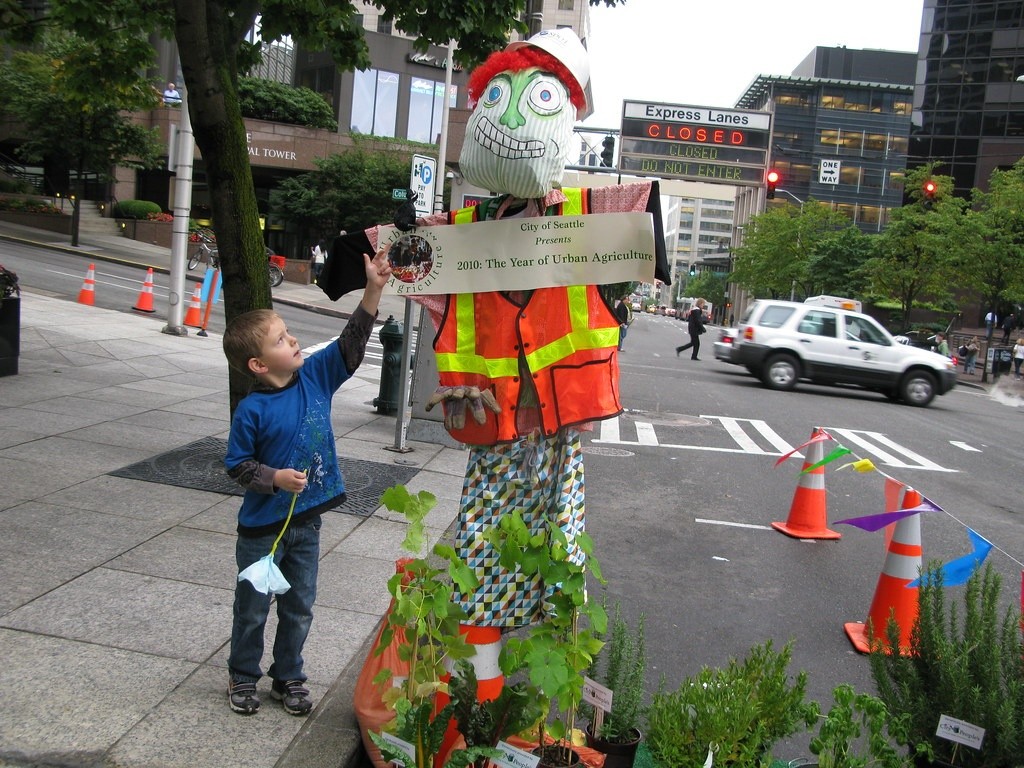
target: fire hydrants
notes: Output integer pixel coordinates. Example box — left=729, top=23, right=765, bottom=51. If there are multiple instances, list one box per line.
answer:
left=373, top=315, right=414, bottom=416
left=724, top=319, right=728, bottom=327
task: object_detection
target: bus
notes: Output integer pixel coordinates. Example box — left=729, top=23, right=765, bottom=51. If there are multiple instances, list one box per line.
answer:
left=674, top=297, right=712, bottom=324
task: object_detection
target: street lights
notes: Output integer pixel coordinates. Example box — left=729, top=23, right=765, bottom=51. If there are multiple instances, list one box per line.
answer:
left=775, top=189, right=804, bottom=302
left=711, top=240, right=731, bottom=326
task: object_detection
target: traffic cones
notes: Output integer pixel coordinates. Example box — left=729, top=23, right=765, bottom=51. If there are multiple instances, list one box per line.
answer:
left=427, top=622, right=504, bottom=768
left=771, top=426, right=842, bottom=539
left=183, top=282, right=203, bottom=328
left=843, top=488, right=923, bottom=657
left=78, top=263, right=157, bottom=313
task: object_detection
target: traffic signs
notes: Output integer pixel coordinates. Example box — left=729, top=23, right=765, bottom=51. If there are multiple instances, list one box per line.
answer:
left=820, top=159, right=840, bottom=185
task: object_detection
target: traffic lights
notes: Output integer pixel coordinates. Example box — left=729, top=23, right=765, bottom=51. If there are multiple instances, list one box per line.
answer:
left=766, top=171, right=777, bottom=199
left=690, top=265, right=695, bottom=276
left=600, top=137, right=614, bottom=167
left=923, top=183, right=934, bottom=210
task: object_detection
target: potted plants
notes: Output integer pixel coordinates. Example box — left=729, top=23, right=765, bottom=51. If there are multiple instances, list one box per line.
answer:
left=482, top=509, right=1024, bottom=768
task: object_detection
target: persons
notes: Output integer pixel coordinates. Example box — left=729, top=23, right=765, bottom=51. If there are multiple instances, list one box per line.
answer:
left=1001, top=314, right=1015, bottom=346
left=962, top=335, right=980, bottom=375
left=163, top=83, right=182, bottom=106
left=676, top=298, right=707, bottom=361
left=730, top=312, right=734, bottom=328
left=1012, top=338, right=1024, bottom=380
left=223, top=248, right=392, bottom=716
left=936, top=332, right=949, bottom=358
left=311, top=239, right=328, bottom=282
left=314, top=27, right=674, bottom=629
left=985, top=312, right=997, bottom=340
left=616, top=296, right=631, bottom=352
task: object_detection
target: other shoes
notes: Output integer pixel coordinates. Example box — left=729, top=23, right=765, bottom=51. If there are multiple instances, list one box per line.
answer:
left=691, top=357, right=701, bottom=360
left=618, top=348, right=624, bottom=352
left=675, top=348, right=680, bottom=356
left=963, top=371, right=967, bottom=374
left=970, top=372, right=974, bottom=375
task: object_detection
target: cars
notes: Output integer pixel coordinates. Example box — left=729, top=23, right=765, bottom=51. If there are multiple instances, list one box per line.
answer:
left=713, top=320, right=862, bottom=364
left=893, top=330, right=938, bottom=352
left=729, top=300, right=957, bottom=407
left=633, top=303, right=676, bottom=317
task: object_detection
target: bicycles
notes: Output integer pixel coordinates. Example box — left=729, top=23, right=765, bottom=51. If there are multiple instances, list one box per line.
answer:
left=188, top=230, right=284, bottom=287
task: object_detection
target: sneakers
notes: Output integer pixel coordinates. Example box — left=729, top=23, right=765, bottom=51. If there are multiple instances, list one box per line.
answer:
left=227, top=674, right=260, bottom=713
left=270, top=678, right=312, bottom=715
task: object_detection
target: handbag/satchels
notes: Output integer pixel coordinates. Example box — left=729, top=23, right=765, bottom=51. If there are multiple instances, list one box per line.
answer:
left=959, top=346, right=968, bottom=357
